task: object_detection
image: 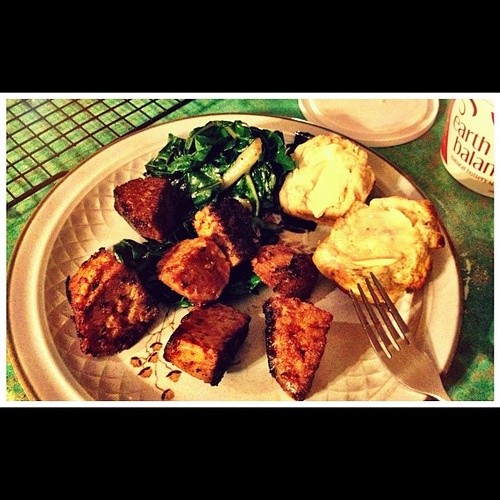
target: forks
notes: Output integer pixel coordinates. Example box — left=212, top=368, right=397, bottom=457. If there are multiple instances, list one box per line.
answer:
left=348, top=271, right=451, bottom=401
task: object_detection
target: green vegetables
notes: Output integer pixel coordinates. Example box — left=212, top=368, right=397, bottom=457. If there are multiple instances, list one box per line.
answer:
left=113, top=120, right=319, bottom=308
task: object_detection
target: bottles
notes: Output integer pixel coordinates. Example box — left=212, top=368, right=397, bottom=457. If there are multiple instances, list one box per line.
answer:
left=439, top=99, right=494, bottom=199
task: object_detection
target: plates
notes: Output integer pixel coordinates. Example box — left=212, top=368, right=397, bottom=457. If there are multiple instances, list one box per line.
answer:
left=8, top=110, right=464, bottom=402
